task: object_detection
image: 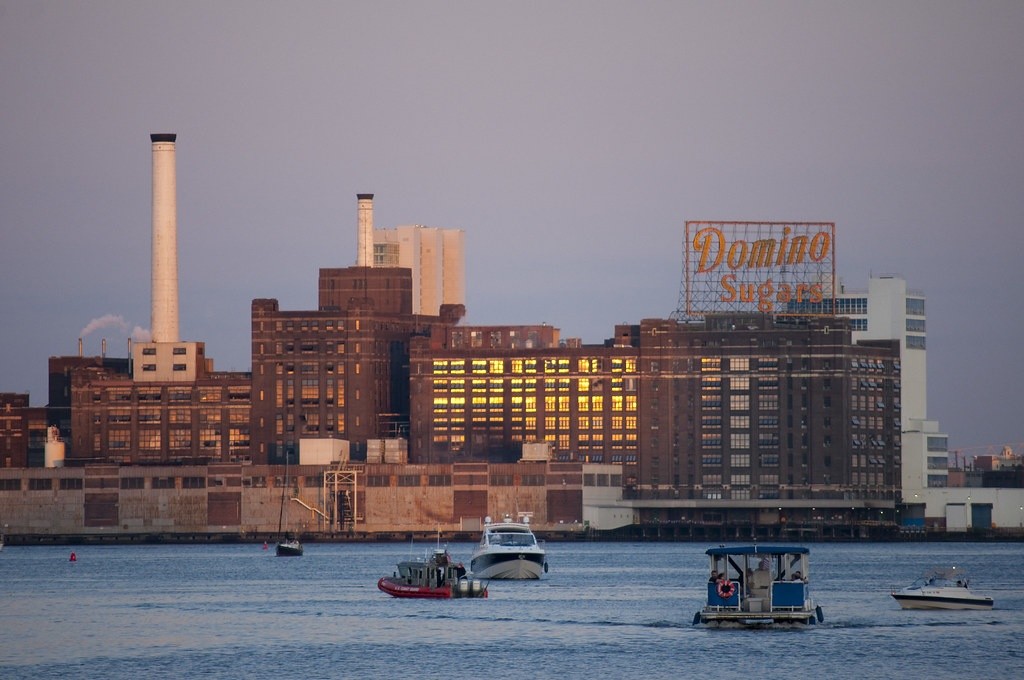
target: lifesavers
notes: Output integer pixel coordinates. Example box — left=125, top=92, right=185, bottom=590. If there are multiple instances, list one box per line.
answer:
left=718, top=580, right=734, bottom=598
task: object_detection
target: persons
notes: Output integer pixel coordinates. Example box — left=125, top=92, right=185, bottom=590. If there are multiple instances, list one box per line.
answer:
left=791, top=571, right=808, bottom=584
left=491, top=531, right=501, bottom=540
left=956, top=581, right=968, bottom=588
left=708, top=571, right=725, bottom=583
left=454, top=562, right=466, bottom=580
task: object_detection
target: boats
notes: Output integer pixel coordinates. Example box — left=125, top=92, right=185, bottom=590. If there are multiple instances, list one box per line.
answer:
left=276, top=539, right=303, bottom=557
left=377, top=544, right=490, bottom=599
left=692, top=540, right=823, bottom=629
left=472, top=510, right=546, bottom=581
left=890, top=563, right=994, bottom=614
